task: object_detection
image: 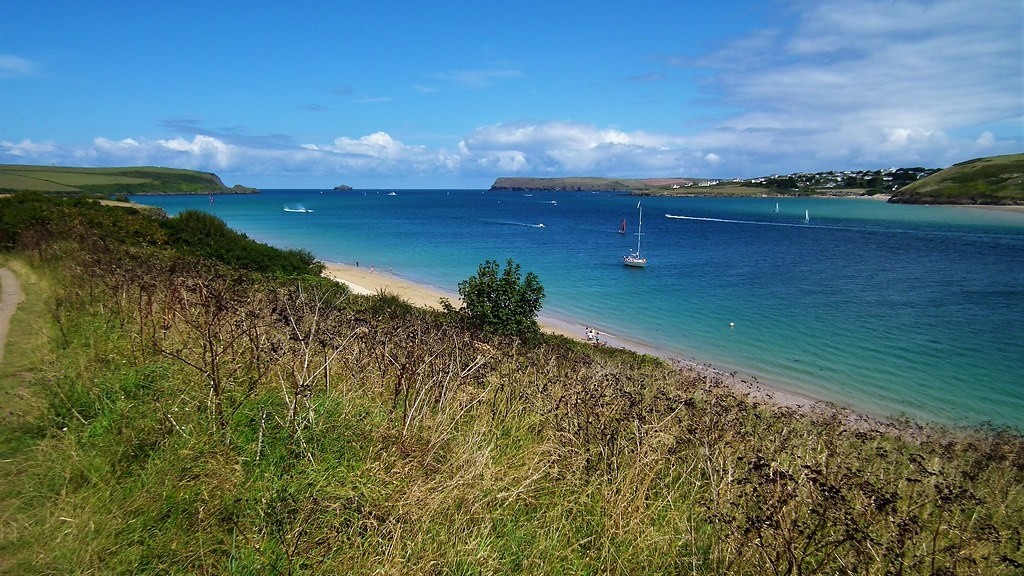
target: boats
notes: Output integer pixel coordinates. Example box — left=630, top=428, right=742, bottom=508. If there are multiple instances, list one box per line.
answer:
left=623, top=255, right=646, bottom=267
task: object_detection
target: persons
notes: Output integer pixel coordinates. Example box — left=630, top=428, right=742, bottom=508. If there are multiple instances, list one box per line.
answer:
left=585, top=325, right=599, bottom=344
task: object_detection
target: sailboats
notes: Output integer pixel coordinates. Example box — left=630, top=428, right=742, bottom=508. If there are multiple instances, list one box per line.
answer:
left=619, top=218, right=626, bottom=234
